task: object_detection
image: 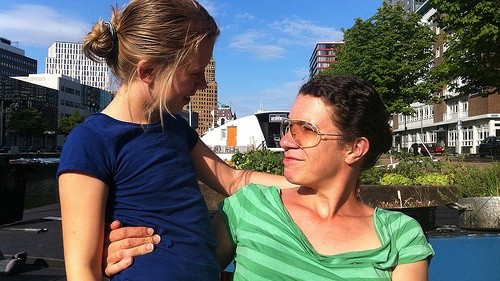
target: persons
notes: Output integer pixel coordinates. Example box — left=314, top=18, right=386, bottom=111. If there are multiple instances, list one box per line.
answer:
left=103, top=74, right=433, bottom=280
left=57, top=1, right=361, bottom=280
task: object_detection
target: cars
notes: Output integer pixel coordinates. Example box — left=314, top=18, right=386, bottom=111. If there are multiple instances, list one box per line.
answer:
left=408, top=144, right=423, bottom=155
left=420, top=143, right=445, bottom=156
left=479, top=136, right=500, bottom=158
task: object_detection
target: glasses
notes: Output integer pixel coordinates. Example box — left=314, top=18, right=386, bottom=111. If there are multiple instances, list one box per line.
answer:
left=280, top=117, right=356, bottom=149
